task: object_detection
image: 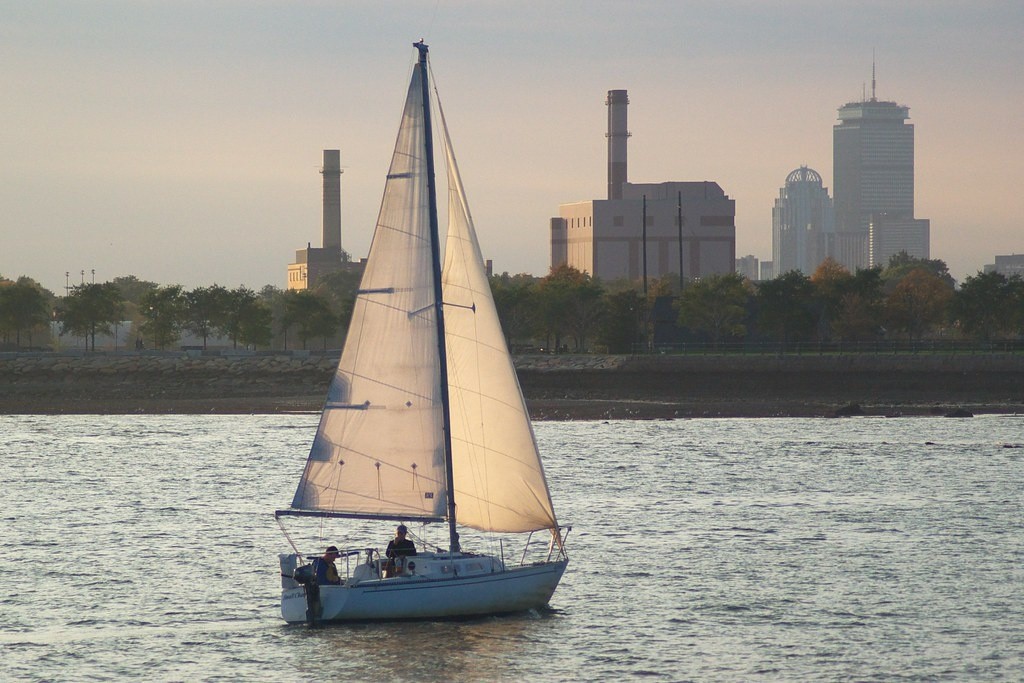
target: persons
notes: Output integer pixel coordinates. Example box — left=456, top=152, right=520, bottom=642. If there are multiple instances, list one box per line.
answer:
left=385, top=524, right=417, bottom=578
left=312, top=546, right=343, bottom=585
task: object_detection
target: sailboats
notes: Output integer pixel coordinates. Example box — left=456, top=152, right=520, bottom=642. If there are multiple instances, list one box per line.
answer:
left=274, top=40, right=579, bottom=623
